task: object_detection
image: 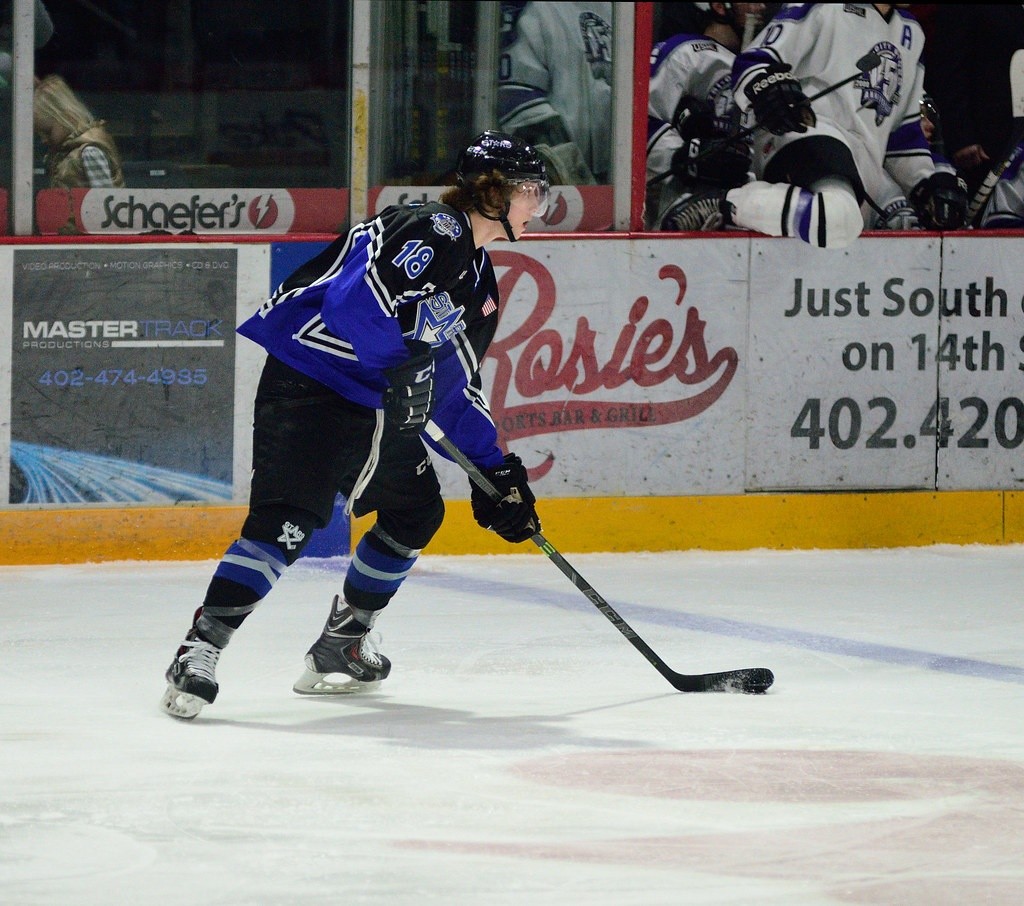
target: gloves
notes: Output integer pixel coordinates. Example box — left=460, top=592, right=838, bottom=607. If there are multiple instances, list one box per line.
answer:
left=382, top=339, right=435, bottom=438
left=670, top=138, right=752, bottom=190
left=466, top=453, right=541, bottom=543
left=744, top=63, right=818, bottom=136
left=908, top=171, right=968, bottom=232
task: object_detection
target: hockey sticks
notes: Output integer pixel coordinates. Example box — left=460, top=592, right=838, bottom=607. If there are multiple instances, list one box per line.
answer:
left=423, top=417, right=777, bottom=695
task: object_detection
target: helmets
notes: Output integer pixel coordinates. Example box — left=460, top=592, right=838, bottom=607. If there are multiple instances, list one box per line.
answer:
left=452, top=130, right=545, bottom=190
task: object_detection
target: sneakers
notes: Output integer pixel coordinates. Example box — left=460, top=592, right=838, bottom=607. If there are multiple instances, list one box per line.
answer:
left=160, top=606, right=222, bottom=719
left=292, top=595, right=391, bottom=695
left=651, top=190, right=727, bottom=232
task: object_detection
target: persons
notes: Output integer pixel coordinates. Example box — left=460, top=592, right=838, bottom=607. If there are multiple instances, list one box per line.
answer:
left=30, top=77, right=125, bottom=191
left=163, top=125, right=553, bottom=723
left=491, top=2, right=1024, bottom=249
